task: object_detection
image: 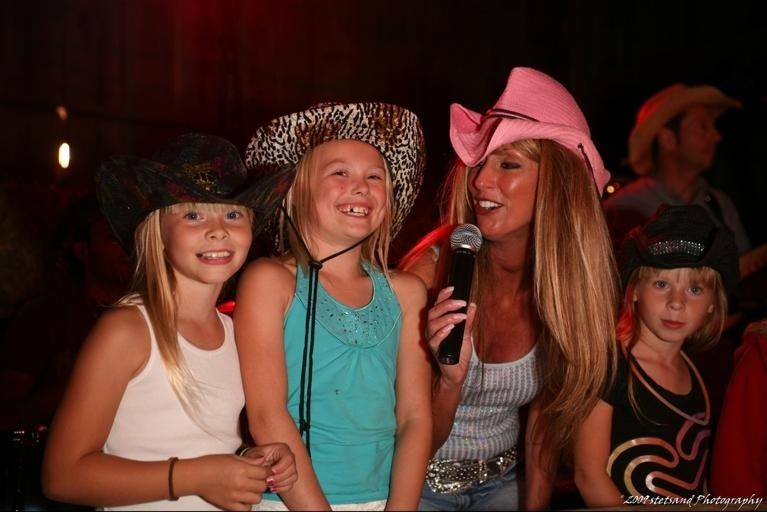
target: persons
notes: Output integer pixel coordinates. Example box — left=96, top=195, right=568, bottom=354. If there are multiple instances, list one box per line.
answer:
left=234, top=100, right=433, bottom=512
left=571, top=202, right=729, bottom=511
left=601, top=82, right=766, bottom=342
left=396, top=65, right=625, bottom=512
left=40, top=138, right=299, bottom=512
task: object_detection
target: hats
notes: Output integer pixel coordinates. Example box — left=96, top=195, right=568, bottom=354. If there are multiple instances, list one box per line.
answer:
left=628, top=83, right=742, bottom=175
left=449, top=68, right=611, bottom=199
left=98, top=134, right=296, bottom=249
left=243, top=103, right=424, bottom=255
left=622, top=202, right=742, bottom=318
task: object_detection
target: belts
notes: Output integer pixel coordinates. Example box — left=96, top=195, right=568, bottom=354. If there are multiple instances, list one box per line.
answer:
left=426, top=446, right=523, bottom=493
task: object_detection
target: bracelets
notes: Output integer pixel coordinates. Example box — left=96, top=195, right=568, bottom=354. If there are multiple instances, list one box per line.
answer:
left=168, top=457, right=179, bottom=502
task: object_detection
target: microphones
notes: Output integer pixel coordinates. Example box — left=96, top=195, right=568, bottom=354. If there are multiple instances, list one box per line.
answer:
left=435, top=223, right=483, bottom=364
